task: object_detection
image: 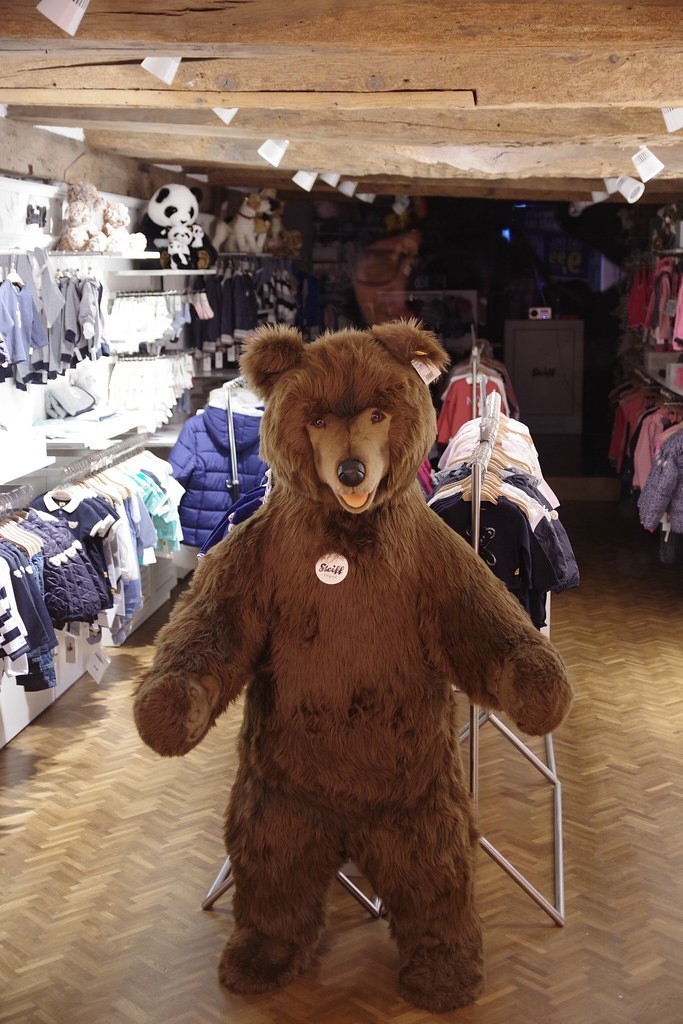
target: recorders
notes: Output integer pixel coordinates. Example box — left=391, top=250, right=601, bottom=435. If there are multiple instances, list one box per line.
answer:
left=527, top=255, right=551, bottom=319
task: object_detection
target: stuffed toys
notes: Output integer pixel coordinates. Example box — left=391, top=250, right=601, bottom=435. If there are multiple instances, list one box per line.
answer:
left=210, top=187, right=313, bottom=257
left=132, top=319, right=572, bottom=1012
left=134, top=184, right=219, bottom=270
left=56, top=181, right=147, bottom=255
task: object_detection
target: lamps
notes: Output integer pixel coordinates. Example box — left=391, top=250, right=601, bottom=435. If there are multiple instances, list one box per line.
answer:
left=338, top=180, right=358, bottom=198
left=392, top=195, right=410, bottom=216
left=320, top=172, right=341, bottom=189
left=355, top=193, right=377, bottom=204
left=140, top=57, right=183, bottom=86
left=257, top=138, right=290, bottom=167
left=290, top=170, right=319, bottom=193
left=660, top=107, right=683, bottom=133
left=615, top=175, right=645, bottom=204
left=603, top=177, right=619, bottom=195
left=35, top=0, right=90, bottom=37
left=591, top=191, right=609, bottom=204
left=631, top=144, right=664, bottom=184
left=210, top=107, right=239, bottom=125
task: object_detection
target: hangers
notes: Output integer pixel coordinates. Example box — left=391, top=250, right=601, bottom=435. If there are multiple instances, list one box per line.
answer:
left=425, top=338, right=536, bottom=524
left=206, top=251, right=317, bottom=289
left=607, top=366, right=683, bottom=459
left=181, top=373, right=267, bottom=432
left=0, top=248, right=103, bottom=301
left=618, top=242, right=683, bottom=289
left=0, top=432, right=175, bottom=575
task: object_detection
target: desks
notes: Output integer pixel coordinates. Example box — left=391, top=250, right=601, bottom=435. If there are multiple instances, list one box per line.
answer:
left=503, top=318, right=582, bottom=436
